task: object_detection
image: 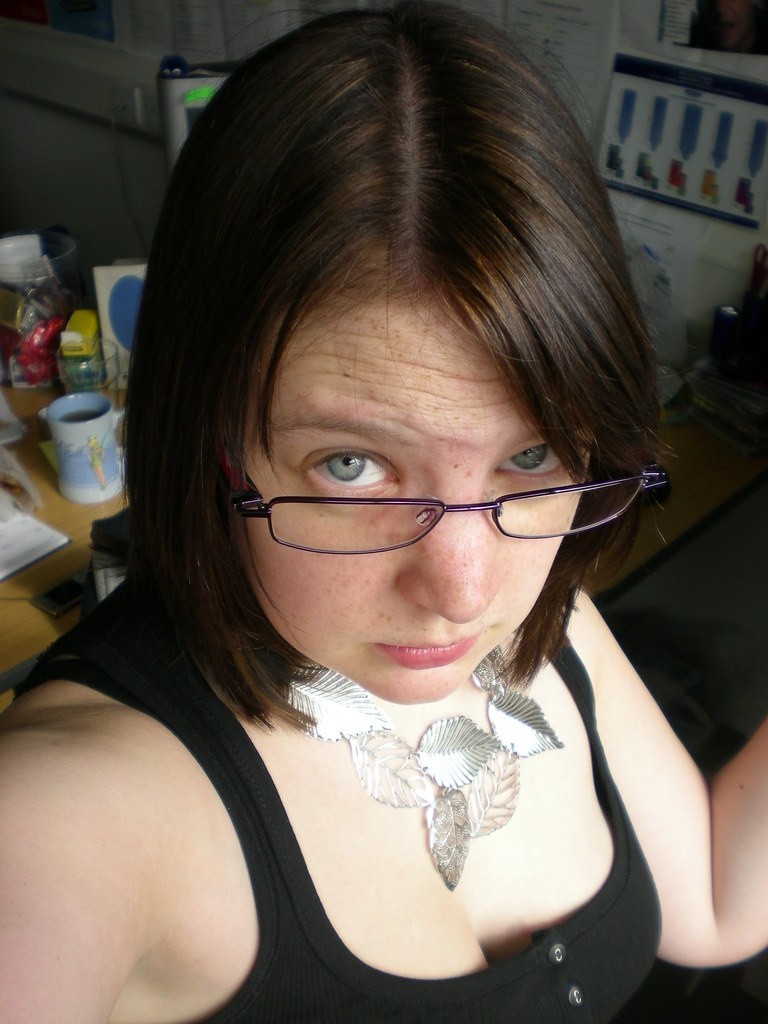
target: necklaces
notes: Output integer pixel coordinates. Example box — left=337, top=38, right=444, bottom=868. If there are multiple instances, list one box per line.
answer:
left=283, top=647, right=565, bottom=893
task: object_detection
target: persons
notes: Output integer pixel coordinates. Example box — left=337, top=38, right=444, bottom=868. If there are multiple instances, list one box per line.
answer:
left=0, top=0, right=768, bottom=1024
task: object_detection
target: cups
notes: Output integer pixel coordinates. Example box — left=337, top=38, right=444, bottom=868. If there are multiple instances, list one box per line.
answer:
left=44, top=393, right=131, bottom=502
left=54, top=337, right=121, bottom=400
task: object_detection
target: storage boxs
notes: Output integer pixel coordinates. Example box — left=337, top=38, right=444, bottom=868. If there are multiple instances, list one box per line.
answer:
left=63, top=308, right=106, bottom=387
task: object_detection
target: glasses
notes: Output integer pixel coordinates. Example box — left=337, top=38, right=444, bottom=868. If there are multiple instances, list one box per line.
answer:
left=213, top=440, right=667, bottom=555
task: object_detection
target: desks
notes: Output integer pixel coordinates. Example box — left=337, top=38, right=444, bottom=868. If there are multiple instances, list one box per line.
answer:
left=1, top=373, right=768, bottom=710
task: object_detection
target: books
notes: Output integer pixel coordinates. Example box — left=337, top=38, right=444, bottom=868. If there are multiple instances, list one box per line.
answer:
left=0, top=485, right=71, bottom=581
